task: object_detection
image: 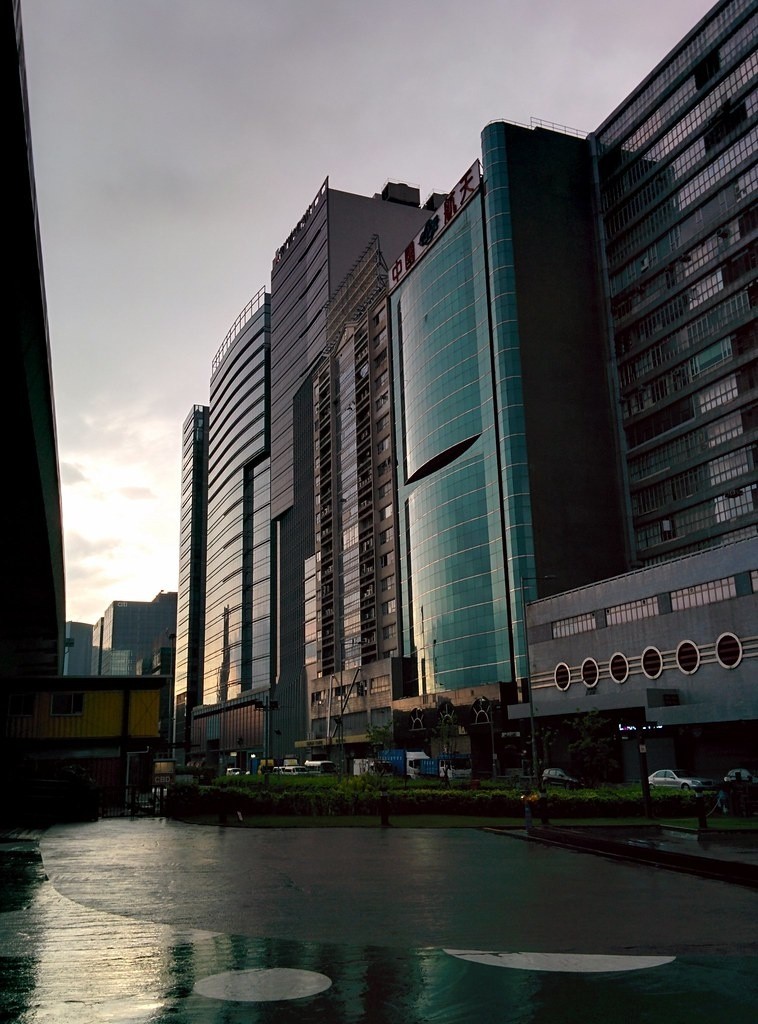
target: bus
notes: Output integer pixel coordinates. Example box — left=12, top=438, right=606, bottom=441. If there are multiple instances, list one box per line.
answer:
left=272, top=766, right=308, bottom=776
left=305, top=760, right=337, bottom=774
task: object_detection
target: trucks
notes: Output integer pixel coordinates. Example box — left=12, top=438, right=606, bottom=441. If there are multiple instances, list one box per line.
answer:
left=419, top=753, right=473, bottom=780
left=378, top=749, right=430, bottom=780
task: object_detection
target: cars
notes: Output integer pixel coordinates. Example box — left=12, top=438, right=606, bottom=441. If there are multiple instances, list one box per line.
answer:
left=723, top=767, right=758, bottom=783
left=541, top=768, right=594, bottom=792
left=648, top=769, right=715, bottom=792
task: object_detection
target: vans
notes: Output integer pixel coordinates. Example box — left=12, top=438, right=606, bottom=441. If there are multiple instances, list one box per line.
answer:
left=226, top=768, right=241, bottom=777
